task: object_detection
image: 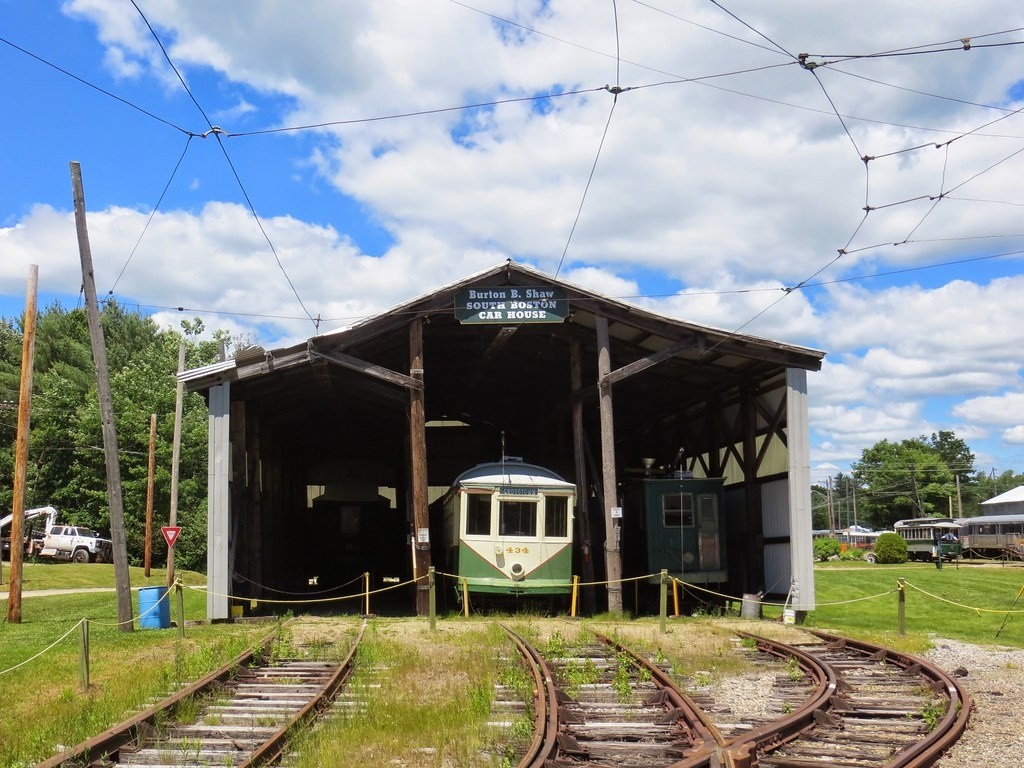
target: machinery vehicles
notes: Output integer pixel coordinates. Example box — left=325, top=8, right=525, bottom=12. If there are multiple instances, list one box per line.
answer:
left=0, top=507, right=59, bottom=536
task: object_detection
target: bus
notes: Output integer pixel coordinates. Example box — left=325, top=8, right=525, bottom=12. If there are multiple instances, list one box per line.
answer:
left=813, top=526, right=893, bottom=564
left=892, top=516, right=962, bottom=564
left=955, top=515, right=1024, bottom=563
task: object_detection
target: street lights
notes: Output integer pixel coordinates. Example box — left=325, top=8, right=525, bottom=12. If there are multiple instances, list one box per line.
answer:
left=811, top=489, right=836, bottom=538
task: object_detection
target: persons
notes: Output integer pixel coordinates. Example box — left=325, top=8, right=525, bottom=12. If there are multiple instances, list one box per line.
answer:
left=941, top=528, right=958, bottom=541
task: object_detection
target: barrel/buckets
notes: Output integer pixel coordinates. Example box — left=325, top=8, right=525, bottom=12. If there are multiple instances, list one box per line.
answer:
left=782, top=609, right=796, bottom=626
left=741, top=594, right=762, bottom=619
left=231, top=606, right=243, bottom=619
left=138, top=586, right=170, bottom=629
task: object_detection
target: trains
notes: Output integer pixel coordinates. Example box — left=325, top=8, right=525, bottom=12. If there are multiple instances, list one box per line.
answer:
left=427, top=433, right=577, bottom=606
left=617, top=447, right=727, bottom=617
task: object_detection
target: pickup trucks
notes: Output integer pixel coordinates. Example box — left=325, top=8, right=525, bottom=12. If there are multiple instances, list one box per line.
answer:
left=0, top=526, right=113, bottom=565
